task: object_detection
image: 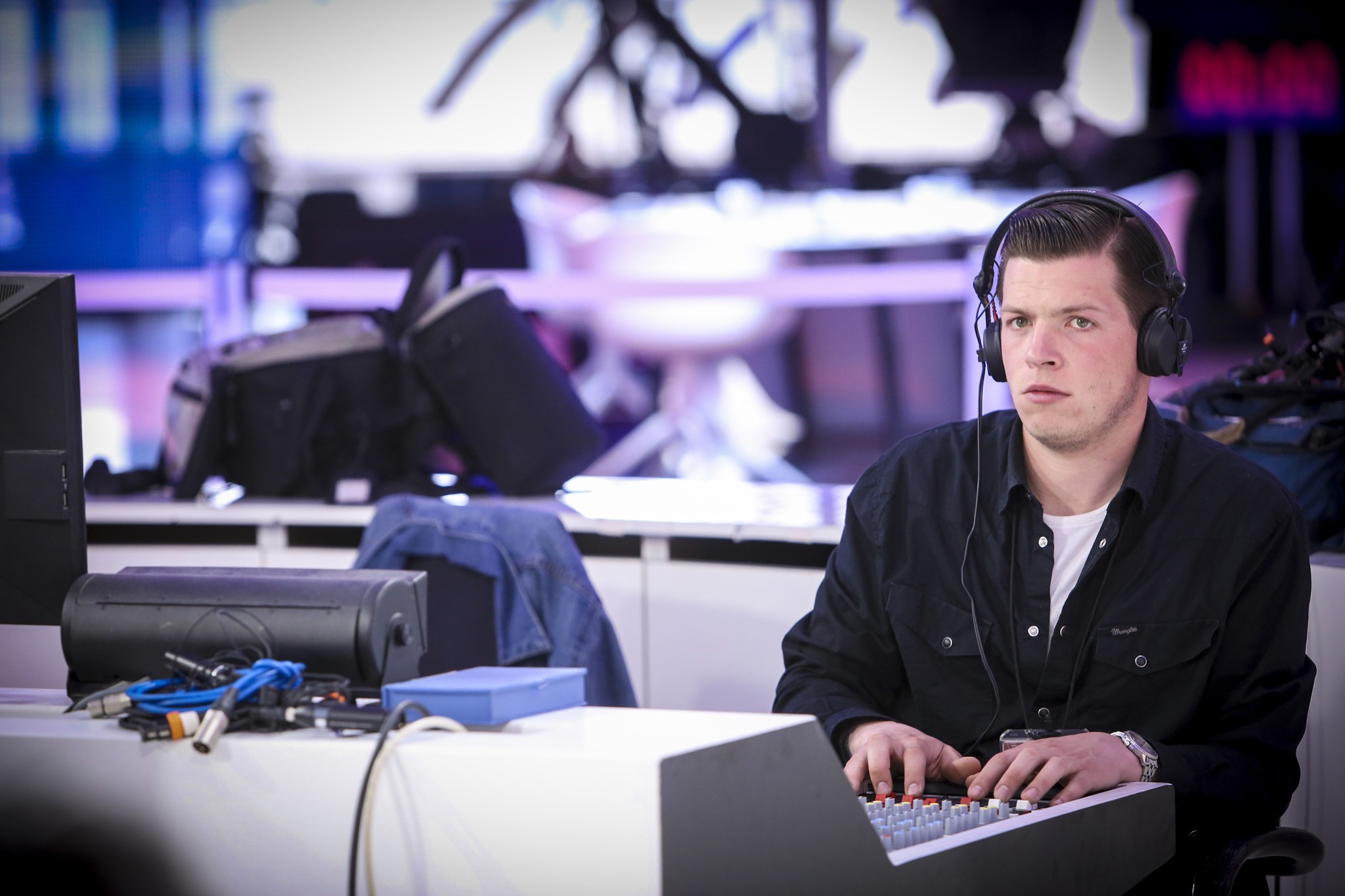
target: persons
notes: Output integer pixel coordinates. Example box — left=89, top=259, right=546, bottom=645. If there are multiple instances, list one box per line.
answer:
left=235, top=88, right=273, bottom=232
left=772, top=186, right=1316, bottom=896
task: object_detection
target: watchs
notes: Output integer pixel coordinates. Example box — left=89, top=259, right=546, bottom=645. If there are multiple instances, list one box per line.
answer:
left=1111, top=730, right=1158, bottom=783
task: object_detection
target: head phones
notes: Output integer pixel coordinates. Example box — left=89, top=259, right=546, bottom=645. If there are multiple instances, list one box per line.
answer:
left=970, top=186, right=1190, bottom=377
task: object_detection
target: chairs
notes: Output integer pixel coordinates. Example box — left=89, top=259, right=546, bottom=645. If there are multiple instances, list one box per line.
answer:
left=350, top=494, right=637, bottom=708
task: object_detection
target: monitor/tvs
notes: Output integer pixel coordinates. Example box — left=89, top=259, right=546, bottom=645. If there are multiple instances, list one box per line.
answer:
left=0, top=269, right=99, bottom=621
